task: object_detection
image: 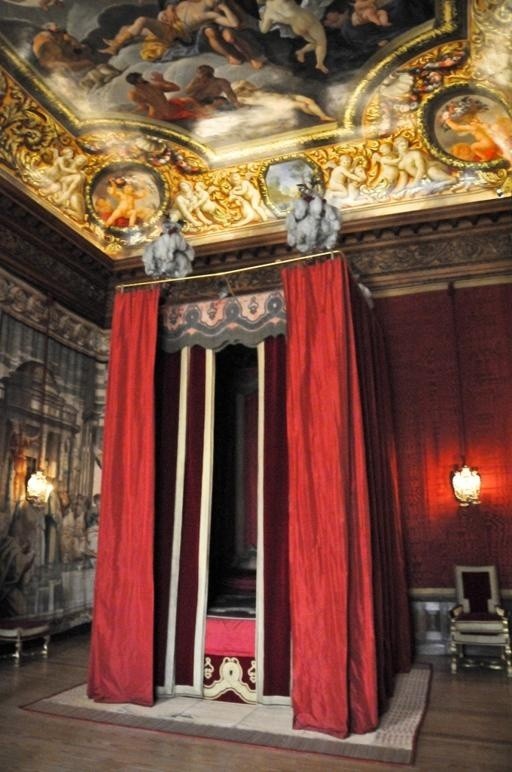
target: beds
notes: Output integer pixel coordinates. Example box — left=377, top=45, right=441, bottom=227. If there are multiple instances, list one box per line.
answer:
left=204, top=595, right=256, bottom=702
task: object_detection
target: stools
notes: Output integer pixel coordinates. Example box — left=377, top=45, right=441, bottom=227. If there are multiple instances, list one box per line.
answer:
left=1, top=620, right=50, bottom=668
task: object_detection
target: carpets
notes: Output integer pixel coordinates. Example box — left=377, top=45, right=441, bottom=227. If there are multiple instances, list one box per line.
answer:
left=16, top=660, right=434, bottom=768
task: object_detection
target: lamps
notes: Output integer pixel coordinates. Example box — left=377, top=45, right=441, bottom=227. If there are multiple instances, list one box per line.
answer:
left=446, top=281, right=482, bottom=507
left=26, top=286, right=55, bottom=507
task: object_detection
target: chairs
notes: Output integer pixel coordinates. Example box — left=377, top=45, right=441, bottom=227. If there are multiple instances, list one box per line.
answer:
left=446, top=564, right=511, bottom=679
